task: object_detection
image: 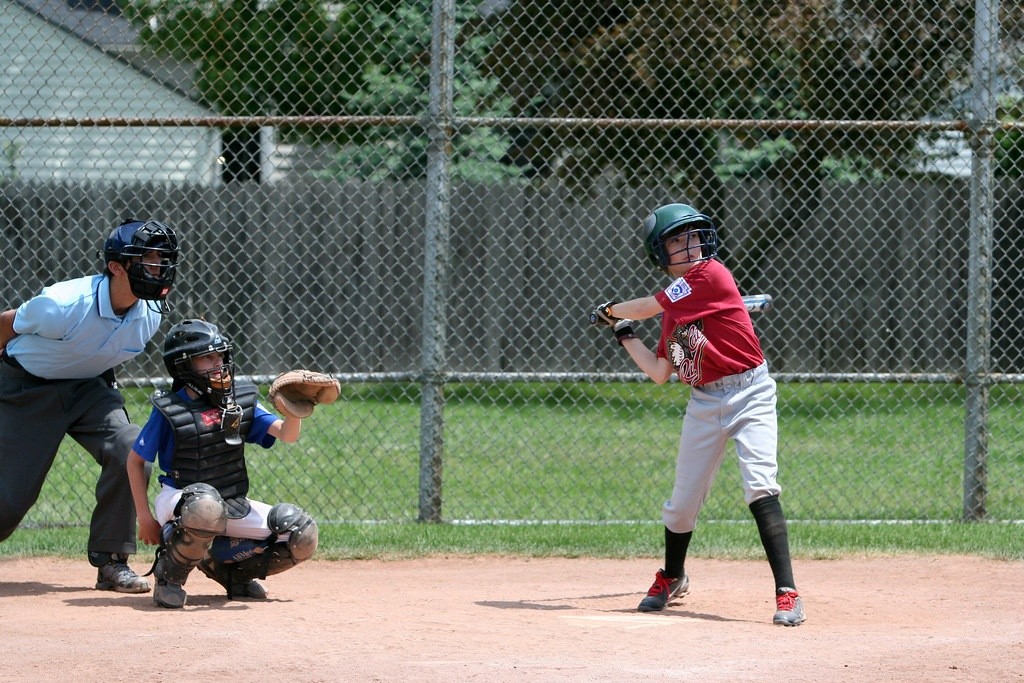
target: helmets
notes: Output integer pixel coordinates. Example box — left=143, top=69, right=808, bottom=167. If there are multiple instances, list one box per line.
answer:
left=103, top=217, right=181, bottom=301
left=640, top=202, right=718, bottom=271
left=162, top=318, right=235, bottom=407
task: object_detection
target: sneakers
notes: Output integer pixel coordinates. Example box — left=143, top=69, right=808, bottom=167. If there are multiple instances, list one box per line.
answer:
left=637, top=568, right=692, bottom=612
left=195, top=547, right=267, bottom=599
left=141, top=546, right=192, bottom=608
left=772, top=587, right=807, bottom=627
left=94, top=558, right=153, bottom=593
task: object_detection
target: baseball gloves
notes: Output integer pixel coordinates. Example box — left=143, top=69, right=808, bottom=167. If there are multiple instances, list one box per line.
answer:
left=267, top=368, right=342, bottom=420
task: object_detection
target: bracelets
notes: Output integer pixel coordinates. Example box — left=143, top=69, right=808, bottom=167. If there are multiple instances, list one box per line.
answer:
left=605, top=302, right=616, bottom=318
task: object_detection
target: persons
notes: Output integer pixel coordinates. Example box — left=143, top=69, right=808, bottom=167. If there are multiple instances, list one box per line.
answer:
left=127, top=319, right=341, bottom=608
left=594, top=203, right=806, bottom=627
left=0, top=217, right=178, bottom=593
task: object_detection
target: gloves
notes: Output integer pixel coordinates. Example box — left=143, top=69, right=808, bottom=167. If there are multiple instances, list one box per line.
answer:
left=590, top=300, right=622, bottom=328
left=611, top=319, right=638, bottom=346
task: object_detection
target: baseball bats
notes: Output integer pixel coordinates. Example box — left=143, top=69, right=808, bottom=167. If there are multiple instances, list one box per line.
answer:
left=588, top=292, right=771, bottom=327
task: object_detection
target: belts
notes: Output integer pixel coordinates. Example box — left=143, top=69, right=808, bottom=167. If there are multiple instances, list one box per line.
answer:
left=1, top=349, right=22, bottom=369
